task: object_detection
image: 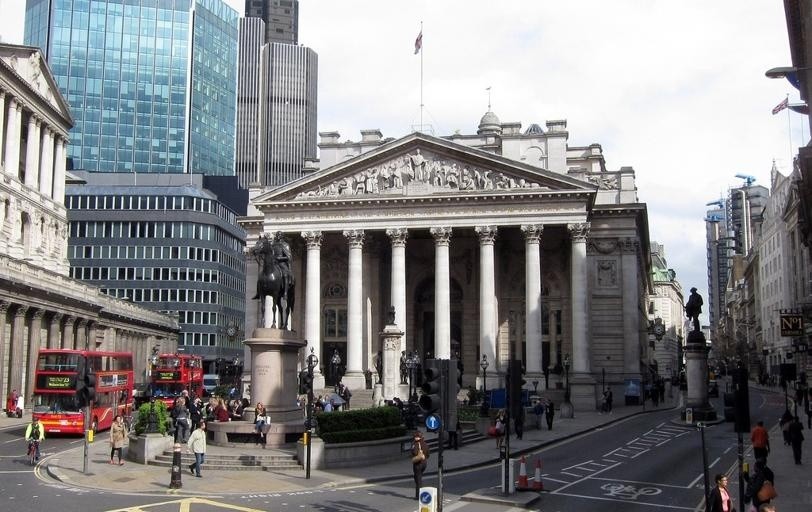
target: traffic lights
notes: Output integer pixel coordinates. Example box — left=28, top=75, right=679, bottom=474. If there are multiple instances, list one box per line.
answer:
left=298, top=372, right=313, bottom=395
left=419, top=359, right=444, bottom=415
left=82, top=373, right=97, bottom=403
left=446, top=359, right=462, bottom=432
left=722, top=367, right=752, bottom=433
left=508, top=360, right=527, bottom=419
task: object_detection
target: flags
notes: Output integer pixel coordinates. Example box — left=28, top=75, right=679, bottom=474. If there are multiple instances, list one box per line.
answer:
left=415, top=31, right=421, bottom=53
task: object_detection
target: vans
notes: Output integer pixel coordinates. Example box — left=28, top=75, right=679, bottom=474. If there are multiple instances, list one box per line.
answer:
left=202, top=374, right=221, bottom=397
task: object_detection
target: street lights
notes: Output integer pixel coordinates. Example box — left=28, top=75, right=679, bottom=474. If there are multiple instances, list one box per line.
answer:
left=404, top=357, right=420, bottom=430
left=329, top=350, right=342, bottom=393
left=562, top=359, right=571, bottom=402
left=531, top=380, right=539, bottom=395
left=480, top=354, right=490, bottom=404
left=147, top=354, right=161, bottom=434
left=412, top=349, right=421, bottom=402
left=306, top=346, right=319, bottom=478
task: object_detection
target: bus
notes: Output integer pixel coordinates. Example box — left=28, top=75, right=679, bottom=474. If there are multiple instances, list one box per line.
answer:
left=32, top=349, right=134, bottom=437
left=151, top=353, right=204, bottom=410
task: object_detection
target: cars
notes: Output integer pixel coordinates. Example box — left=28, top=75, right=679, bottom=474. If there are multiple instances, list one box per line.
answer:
left=131, top=383, right=150, bottom=410
left=678, top=370, right=720, bottom=398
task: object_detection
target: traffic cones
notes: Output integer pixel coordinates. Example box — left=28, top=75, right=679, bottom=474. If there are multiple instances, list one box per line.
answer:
left=533, top=456, right=544, bottom=490
left=518, top=454, right=530, bottom=489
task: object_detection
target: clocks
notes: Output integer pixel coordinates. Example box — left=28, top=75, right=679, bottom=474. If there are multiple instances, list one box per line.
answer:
left=648, top=316, right=666, bottom=342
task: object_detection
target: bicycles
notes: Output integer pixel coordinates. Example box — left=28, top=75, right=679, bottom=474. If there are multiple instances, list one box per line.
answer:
left=27, top=438, right=39, bottom=465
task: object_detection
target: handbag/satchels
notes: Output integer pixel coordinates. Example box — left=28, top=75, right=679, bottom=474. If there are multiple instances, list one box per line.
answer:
left=412, top=451, right=425, bottom=463
left=758, top=481, right=776, bottom=501
left=495, top=421, right=504, bottom=433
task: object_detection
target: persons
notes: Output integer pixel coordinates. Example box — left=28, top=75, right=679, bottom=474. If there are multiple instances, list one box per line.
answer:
left=4, top=382, right=269, bottom=465
left=249, top=231, right=295, bottom=301
left=297, top=382, right=557, bottom=450
left=188, top=419, right=207, bottom=476
left=296, top=149, right=508, bottom=194
left=376, top=350, right=383, bottom=384
left=411, top=428, right=430, bottom=499
left=648, top=355, right=810, bottom=512
left=596, top=385, right=616, bottom=416
left=684, top=286, right=704, bottom=333
left=400, top=351, right=409, bottom=385
